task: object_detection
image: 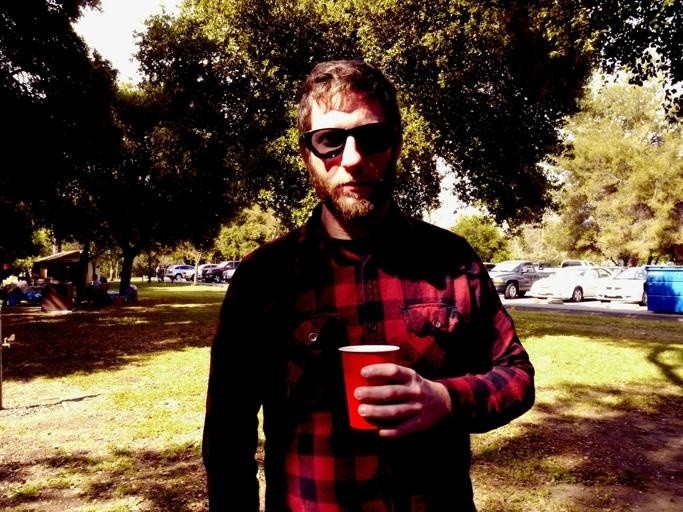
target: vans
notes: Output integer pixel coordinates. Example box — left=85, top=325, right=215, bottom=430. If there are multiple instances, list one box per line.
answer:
left=165, top=260, right=242, bottom=281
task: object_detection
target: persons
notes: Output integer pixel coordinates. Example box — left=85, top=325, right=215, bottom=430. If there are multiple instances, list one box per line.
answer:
left=100, top=277, right=107, bottom=286
left=87, top=273, right=102, bottom=306
left=74, top=272, right=89, bottom=305
left=204, top=60, right=537, bottom=511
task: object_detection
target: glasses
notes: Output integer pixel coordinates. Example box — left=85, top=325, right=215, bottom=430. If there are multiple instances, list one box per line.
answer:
left=298, top=121, right=395, bottom=159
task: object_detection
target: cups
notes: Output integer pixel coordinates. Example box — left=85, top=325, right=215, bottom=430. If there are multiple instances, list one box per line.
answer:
left=338, top=345, right=402, bottom=432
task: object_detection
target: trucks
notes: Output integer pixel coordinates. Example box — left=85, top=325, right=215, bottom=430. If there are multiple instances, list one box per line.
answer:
left=488, top=260, right=556, bottom=300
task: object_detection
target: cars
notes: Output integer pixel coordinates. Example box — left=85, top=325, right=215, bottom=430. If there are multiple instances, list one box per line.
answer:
left=529, top=265, right=618, bottom=303
left=595, top=266, right=648, bottom=307
